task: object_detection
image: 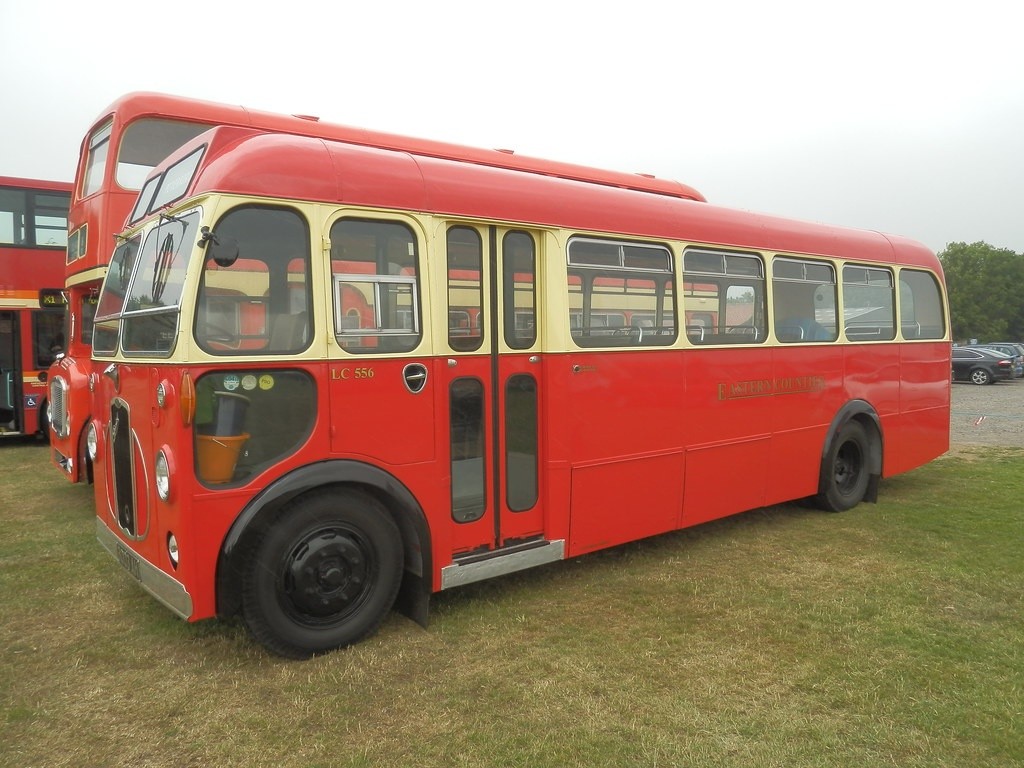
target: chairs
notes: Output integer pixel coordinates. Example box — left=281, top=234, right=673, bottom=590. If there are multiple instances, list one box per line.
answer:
left=259, top=310, right=807, bottom=451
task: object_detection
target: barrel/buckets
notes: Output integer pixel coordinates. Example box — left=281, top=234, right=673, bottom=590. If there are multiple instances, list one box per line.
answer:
left=210, top=390, right=251, bottom=437
left=196, top=433, right=249, bottom=484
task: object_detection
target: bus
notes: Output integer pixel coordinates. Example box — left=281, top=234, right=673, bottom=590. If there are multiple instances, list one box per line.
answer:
left=87, top=125, right=952, bottom=661
left=45, top=89, right=725, bottom=485
left=0, top=175, right=78, bottom=440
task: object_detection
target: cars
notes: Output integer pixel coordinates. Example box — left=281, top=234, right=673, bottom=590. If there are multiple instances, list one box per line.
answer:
left=952, top=341, right=1024, bottom=386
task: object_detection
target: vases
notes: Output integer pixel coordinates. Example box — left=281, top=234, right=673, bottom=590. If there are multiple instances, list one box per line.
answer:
left=194, top=427, right=253, bottom=485
